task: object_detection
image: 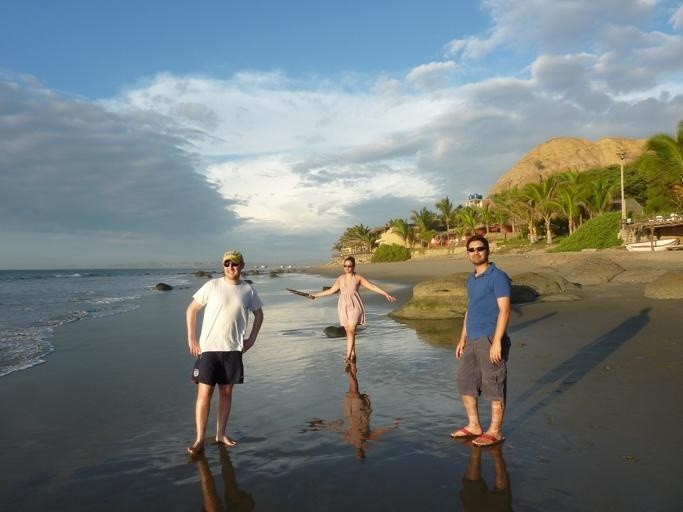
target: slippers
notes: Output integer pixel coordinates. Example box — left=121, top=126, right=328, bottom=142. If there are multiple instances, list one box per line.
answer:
left=451, top=427, right=483, bottom=438
left=472, top=433, right=506, bottom=447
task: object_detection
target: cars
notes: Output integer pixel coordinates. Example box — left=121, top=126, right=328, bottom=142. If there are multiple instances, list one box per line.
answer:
left=527, top=231, right=555, bottom=240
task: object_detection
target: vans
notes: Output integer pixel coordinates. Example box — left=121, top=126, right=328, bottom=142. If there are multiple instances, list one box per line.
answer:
left=656, top=216, right=663, bottom=222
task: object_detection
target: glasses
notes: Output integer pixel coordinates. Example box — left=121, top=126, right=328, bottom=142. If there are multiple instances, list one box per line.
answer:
left=344, top=265, right=351, bottom=268
left=468, top=247, right=486, bottom=252
left=224, top=262, right=238, bottom=267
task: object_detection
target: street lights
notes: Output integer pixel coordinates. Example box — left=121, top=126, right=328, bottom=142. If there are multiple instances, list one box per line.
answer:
left=616, top=147, right=627, bottom=219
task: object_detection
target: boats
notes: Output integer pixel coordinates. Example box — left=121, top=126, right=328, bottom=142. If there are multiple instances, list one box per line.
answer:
left=625, top=239, right=679, bottom=251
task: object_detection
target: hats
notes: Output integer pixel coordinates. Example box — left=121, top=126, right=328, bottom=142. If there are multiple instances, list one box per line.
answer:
left=222, top=250, right=243, bottom=264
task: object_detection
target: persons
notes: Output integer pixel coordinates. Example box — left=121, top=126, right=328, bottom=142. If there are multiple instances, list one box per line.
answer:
left=186, top=250, right=263, bottom=454
left=450, top=236, right=511, bottom=447
left=309, top=257, right=397, bottom=362
